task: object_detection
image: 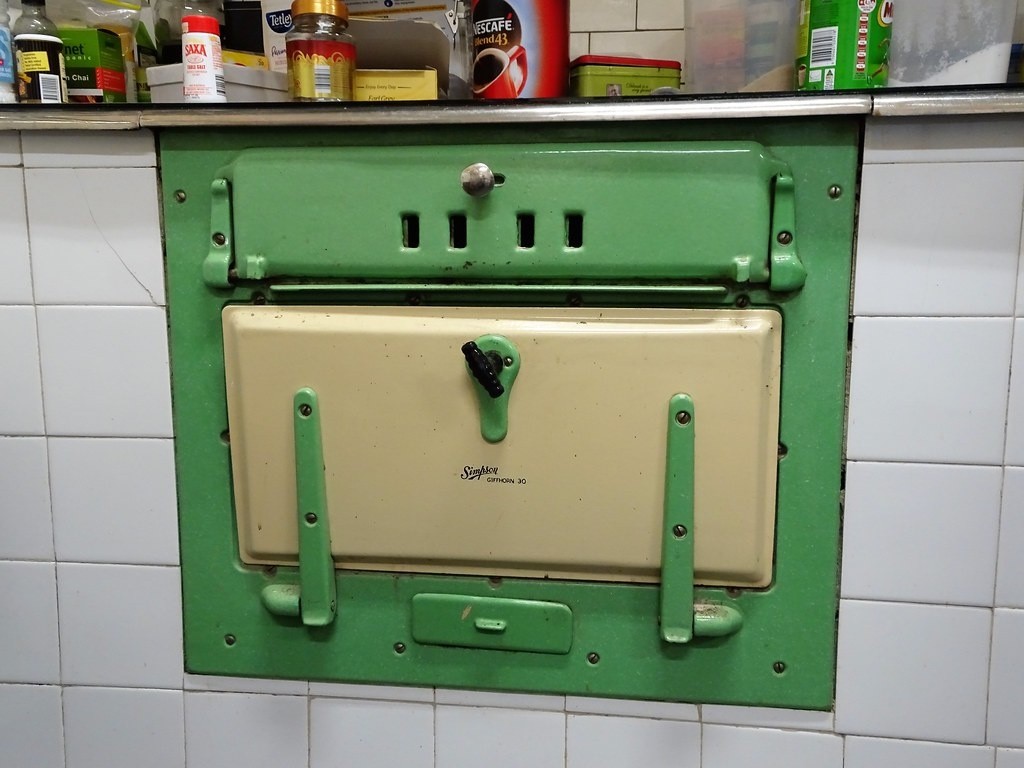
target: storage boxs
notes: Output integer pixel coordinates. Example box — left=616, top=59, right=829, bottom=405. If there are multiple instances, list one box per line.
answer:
left=684, top=0, right=1016, bottom=94
left=145, top=64, right=286, bottom=103
left=57, top=27, right=127, bottom=103
left=353, top=68, right=437, bottom=99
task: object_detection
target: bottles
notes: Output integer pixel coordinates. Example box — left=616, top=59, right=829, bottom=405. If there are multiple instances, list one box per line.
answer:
left=0, top=0, right=69, bottom=104
left=286, top=0, right=356, bottom=102
